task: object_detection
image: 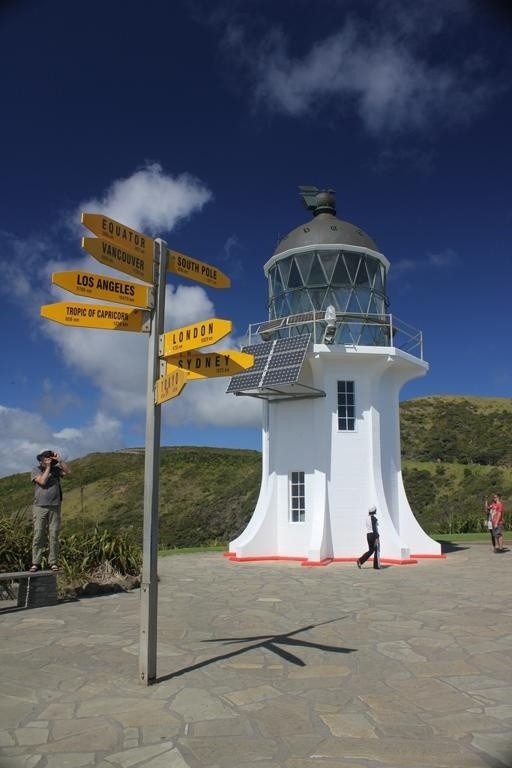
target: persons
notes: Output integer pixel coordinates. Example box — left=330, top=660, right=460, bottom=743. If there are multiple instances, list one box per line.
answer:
left=485, top=499, right=495, bottom=546
left=356, top=506, right=382, bottom=569
left=29, top=449, right=72, bottom=572
left=485, top=493, right=505, bottom=553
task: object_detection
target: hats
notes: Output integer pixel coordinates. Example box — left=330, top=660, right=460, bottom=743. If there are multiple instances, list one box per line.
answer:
left=37, top=449, right=58, bottom=466
left=368, top=505, right=376, bottom=513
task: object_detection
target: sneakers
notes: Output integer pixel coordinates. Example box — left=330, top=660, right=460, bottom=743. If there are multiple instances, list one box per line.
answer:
left=51, top=564, right=59, bottom=570
left=30, top=564, right=38, bottom=571
left=357, top=559, right=362, bottom=569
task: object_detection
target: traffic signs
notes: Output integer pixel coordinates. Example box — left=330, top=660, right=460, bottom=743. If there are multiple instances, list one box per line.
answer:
left=153, top=367, right=189, bottom=406
left=81, top=211, right=155, bottom=260
left=162, top=317, right=232, bottom=356
left=166, top=350, right=256, bottom=380
left=52, top=268, right=156, bottom=311
left=163, top=348, right=203, bottom=362
left=82, top=236, right=154, bottom=282
left=41, top=301, right=151, bottom=334
left=167, top=249, right=232, bottom=289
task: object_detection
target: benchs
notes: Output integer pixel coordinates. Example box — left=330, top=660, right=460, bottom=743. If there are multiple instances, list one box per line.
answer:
left=0, top=567, right=63, bottom=613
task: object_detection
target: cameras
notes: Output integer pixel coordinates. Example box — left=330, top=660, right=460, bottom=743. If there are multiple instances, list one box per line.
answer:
left=50, top=459, right=58, bottom=466
